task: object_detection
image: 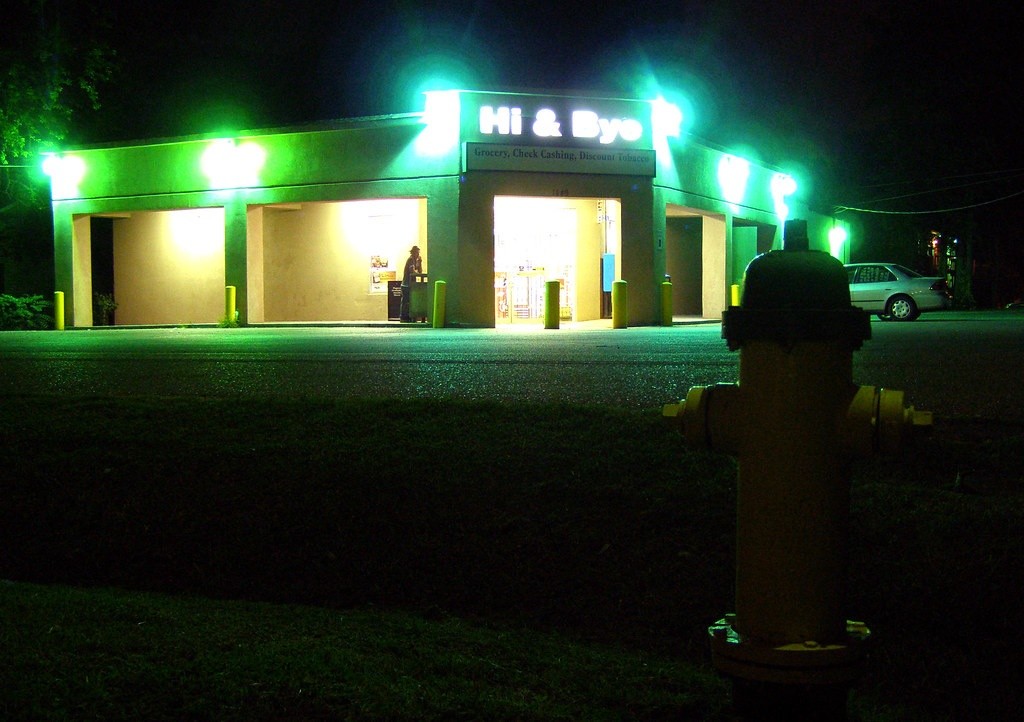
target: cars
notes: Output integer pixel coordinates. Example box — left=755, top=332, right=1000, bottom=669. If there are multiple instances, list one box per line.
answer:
left=843, top=261, right=953, bottom=320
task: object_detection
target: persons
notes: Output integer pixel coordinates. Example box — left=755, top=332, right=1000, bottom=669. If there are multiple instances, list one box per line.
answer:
left=399, top=245, right=423, bottom=323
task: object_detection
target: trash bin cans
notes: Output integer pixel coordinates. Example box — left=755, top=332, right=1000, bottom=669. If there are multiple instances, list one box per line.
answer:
left=408, top=274, right=429, bottom=323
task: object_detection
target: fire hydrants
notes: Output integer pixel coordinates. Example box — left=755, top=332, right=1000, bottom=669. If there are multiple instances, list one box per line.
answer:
left=661, top=218, right=934, bottom=721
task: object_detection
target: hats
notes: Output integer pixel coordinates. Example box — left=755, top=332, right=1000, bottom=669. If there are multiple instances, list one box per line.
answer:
left=410, top=246, right=420, bottom=252
